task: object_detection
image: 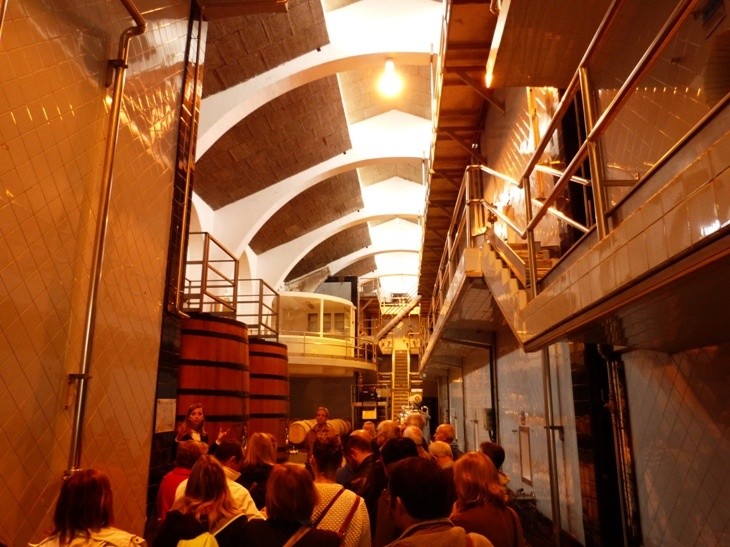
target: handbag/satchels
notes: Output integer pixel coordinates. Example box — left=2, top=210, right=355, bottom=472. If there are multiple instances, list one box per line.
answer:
left=177, top=532, right=219, bottom=547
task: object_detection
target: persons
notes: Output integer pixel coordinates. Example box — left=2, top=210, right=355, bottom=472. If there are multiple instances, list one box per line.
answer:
left=28, top=468, right=148, bottom=547
left=151, top=403, right=525, bottom=547
